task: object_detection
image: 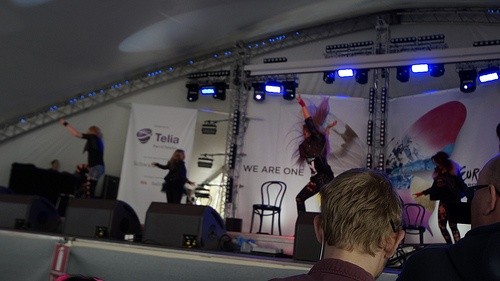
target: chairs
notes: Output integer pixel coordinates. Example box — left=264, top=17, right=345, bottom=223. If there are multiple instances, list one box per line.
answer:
left=249, top=181, right=287, bottom=236
left=402, top=203, right=426, bottom=246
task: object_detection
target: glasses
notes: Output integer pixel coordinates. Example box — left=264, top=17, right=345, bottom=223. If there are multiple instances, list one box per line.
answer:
left=465, top=184, right=488, bottom=198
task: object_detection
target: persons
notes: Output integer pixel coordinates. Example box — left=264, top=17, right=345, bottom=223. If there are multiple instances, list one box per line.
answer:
left=50, top=118, right=105, bottom=198
left=412, top=152, right=466, bottom=245
left=152, top=148, right=195, bottom=204
left=396, top=156, right=500, bottom=281
left=268, top=168, right=405, bottom=281
left=295, top=92, right=338, bottom=215
